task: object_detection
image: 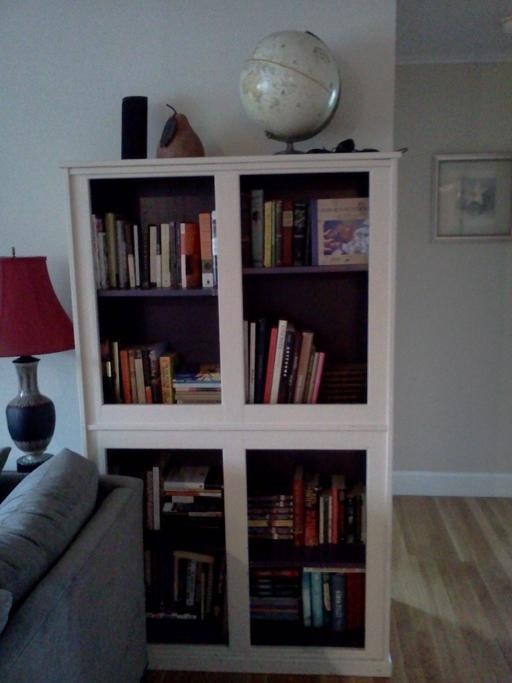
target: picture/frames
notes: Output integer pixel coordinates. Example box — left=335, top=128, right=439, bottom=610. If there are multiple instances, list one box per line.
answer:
left=309, top=191, right=369, bottom=267
left=430, top=152, right=512, bottom=242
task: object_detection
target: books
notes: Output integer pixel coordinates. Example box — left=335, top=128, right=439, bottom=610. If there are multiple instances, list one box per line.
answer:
left=243, top=318, right=366, bottom=404
left=246, top=462, right=368, bottom=633
left=147, top=462, right=228, bottom=632
left=100, top=336, right=221, bottom=404
left=240, top=190, right=368, bottom=268
left=91, top=210, right=218, bottom=290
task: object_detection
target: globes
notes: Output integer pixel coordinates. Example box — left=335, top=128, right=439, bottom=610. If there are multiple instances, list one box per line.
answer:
left=236, top=29, right=341, bottom=154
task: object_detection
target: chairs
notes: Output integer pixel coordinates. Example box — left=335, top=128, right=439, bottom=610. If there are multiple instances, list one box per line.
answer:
left=0, top=471, right=150, bottom=683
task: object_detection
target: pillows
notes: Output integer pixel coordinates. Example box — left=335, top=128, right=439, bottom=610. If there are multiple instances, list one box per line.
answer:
left=0, top=589, right=13, bottom=634
left=0, top=446, right=99, bottom=607
left=0, top=446, right=11, bottom=473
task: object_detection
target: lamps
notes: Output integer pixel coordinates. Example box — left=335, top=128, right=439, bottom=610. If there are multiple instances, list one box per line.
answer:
left=58, top=150, right=403, bottom=676
left=0, top=244, right=76, bottom=474
